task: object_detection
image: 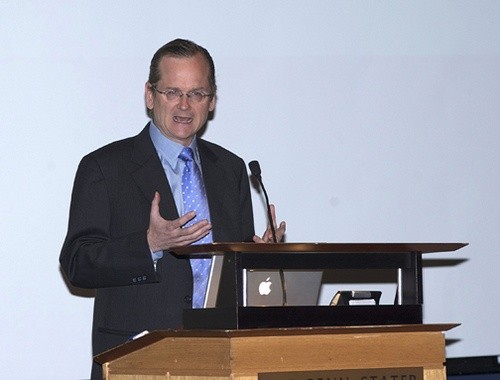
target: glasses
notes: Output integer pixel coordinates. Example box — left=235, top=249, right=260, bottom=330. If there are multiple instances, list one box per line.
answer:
left=151, top=86, right=213, bottom=102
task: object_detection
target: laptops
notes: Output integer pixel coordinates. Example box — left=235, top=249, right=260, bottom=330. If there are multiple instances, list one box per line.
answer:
left=201, top=253, right=324, bottom=307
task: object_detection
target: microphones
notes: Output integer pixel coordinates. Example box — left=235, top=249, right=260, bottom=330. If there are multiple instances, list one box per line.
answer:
left=248, top=159, right=281, bottom=242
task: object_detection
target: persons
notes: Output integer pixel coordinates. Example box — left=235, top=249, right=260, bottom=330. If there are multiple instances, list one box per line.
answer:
left=57, top=39, right=287, bottom=380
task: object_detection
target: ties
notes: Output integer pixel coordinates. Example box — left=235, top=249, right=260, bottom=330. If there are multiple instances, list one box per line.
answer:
left=179, top=148, right=213, bottom=308
